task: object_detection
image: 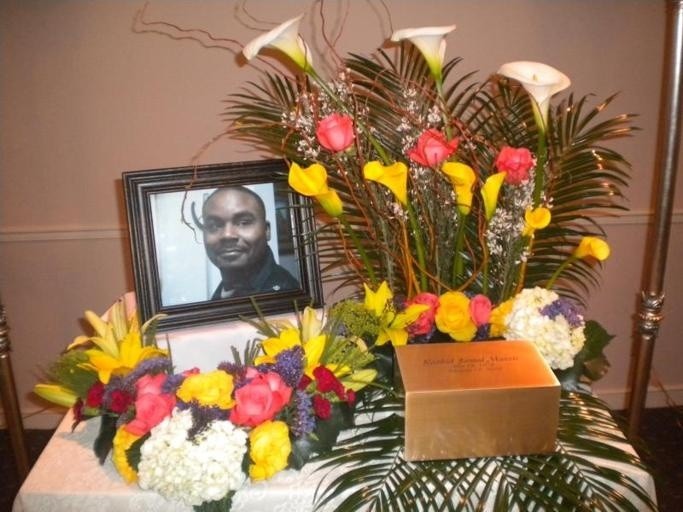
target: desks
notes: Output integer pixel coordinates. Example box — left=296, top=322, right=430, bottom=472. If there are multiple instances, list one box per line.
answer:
left=15, top=372, right=658, bottom=512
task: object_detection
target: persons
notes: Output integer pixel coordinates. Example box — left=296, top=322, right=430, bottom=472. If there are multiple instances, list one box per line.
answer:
left=202, top=185, right=303, bottom=301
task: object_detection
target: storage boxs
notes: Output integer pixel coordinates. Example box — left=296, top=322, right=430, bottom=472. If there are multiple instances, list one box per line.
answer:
left=390, top=339, right=559, bottom=461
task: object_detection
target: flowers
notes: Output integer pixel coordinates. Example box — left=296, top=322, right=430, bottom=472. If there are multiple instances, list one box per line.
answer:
left=235, top=6, right=617, bottom=367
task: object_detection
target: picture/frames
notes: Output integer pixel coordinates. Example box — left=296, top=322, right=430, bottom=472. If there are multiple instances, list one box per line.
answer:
left=121, top=159, right=325, bottom=332
